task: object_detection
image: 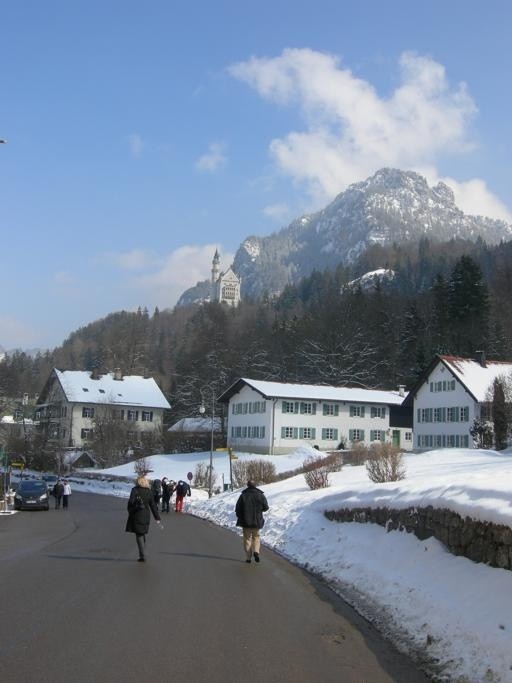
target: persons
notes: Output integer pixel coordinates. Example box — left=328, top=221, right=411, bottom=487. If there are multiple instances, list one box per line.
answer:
left=236, top=480, right=269, bottom=563
left=52, top=479, right=63, bottom=510
left=152, top=477, right=162, bottom=511
left=63, top=480, right=72, bottom=509
left=162, top=477, right=175, bottom=514
left=125, top=476, right=161, bottom=562
left=174, top=480, right=191, bottom=513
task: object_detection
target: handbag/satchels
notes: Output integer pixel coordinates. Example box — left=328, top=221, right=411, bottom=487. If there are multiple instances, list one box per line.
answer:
left=128, top=495, right=145, bottom=513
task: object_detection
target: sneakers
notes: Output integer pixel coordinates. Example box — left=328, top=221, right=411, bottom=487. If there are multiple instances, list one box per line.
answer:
left=254, top=552, right=259, bottom=562
left=138, top=557, right=144, bottom=562
left=247, top=560, right=251, bottom=563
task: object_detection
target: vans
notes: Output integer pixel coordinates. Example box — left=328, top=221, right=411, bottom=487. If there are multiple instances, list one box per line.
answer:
left=42, top=474, right=58, bottom=492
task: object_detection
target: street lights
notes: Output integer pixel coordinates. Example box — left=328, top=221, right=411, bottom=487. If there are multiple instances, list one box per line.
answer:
left=198, top=385, right=215, bottom=499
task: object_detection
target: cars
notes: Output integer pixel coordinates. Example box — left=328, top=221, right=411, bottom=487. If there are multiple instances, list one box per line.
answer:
left=12, top=479, right=49, bottom=510
